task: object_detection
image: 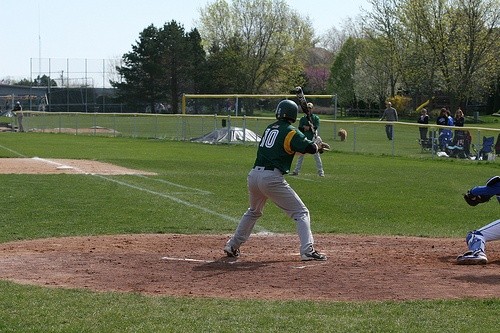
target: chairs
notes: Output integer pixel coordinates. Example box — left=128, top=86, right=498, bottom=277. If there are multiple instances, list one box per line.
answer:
left=416, top=129, right=497, bottom=161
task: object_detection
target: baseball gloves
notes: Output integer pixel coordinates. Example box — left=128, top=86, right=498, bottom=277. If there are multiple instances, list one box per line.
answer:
left=462, top=190, right=492, bottom=207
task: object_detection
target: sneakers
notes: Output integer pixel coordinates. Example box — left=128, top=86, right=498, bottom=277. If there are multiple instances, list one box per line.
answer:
left=225, top=241, right=241, bottom=257
left=300, top=248, right=327, bottom=261
left=456, top=249, right=487, bottom=263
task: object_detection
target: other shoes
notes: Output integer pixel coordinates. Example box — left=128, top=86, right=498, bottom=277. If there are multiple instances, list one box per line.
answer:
left=319, top=172, right=325, bottom=178
left=288, top=172, right=299, bottom=176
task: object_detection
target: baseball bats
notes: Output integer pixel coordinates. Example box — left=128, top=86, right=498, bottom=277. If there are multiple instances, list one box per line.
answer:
left=294, top=85, right=324, bottom=154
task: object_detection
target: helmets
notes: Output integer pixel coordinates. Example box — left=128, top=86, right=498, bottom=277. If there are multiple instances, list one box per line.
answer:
left=275, top=100, right=297, bottom=124
left=15, top=101, right=20, bottom=106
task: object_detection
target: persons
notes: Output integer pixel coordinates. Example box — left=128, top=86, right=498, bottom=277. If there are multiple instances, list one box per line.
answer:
left=224, top=100, right=329, bottom=262
left=381, top=100, right=399, bottom=138
left=457, top=174, right=500, bottom=263
left=14, top=101, right=26, bottom=134
left=289, top=101, right=325, bottom=175
left=437, top=106, right=472, bottom=153
left=417, top=109, right=428, bottom=144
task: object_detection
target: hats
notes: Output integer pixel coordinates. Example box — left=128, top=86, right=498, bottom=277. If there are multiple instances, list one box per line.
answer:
left=305, top=102, right=314, bottom=109
